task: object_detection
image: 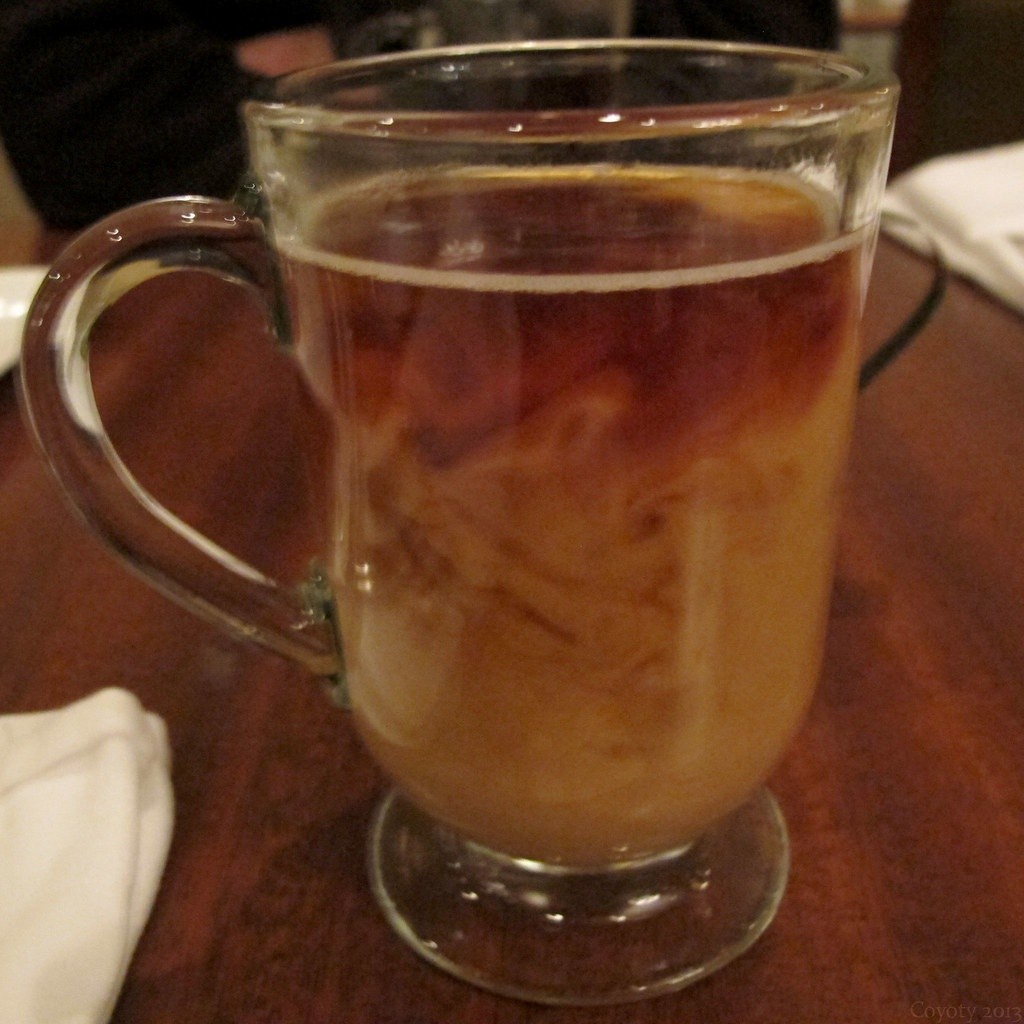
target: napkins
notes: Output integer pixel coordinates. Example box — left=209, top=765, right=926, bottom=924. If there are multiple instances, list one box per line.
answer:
left=1, top=685, right=177, bottom=1024
left=878, top=139, right=1023, bottom=320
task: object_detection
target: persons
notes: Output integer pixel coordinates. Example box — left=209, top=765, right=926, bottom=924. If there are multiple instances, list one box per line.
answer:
left=1, top=0, right=843, bottom=228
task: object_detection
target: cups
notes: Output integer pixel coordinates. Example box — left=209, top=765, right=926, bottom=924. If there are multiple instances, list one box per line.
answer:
left=19, top=40, right=904, bottom=1007
